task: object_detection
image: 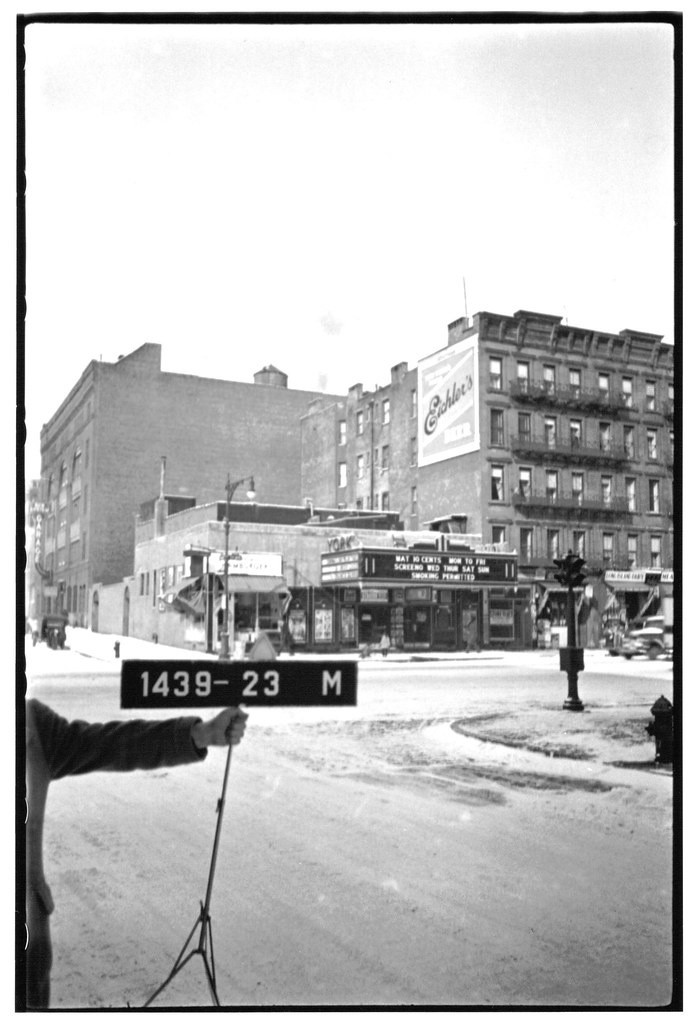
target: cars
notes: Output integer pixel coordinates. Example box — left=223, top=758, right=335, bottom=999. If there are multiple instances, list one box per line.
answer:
left=604, top=613, right=667, bottom=661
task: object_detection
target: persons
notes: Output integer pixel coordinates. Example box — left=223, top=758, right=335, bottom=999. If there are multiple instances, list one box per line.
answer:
left=380, top=632, right=390, bottom=656
left=26, top=697, right=248, bottom=1009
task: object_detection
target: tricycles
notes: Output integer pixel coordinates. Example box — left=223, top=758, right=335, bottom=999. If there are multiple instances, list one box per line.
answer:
left=31, top=613, right=70, bottom=650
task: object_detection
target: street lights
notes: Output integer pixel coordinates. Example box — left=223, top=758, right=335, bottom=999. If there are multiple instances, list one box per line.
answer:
left=219, top=471, right=258, bottom=660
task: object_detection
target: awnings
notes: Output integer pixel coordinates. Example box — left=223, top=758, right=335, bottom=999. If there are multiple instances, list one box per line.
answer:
left=543, top=583, right=584, bottom=591
left=606, top=580, right=650, bottom=591
left=164, top=576, right=201, bottom=595
left=220, top=575, right=288, bottom=593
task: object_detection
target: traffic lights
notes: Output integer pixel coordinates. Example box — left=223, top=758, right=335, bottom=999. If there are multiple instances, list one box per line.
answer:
left=567, top=554, right=587, bottom=587
left=552, top=558, right=567, bottom=586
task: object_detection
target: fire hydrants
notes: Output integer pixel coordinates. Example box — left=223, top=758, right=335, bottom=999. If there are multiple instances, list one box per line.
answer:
left=643, top=696, right=673, bottom=766
left=113, top=641, right=121, bottom=658
